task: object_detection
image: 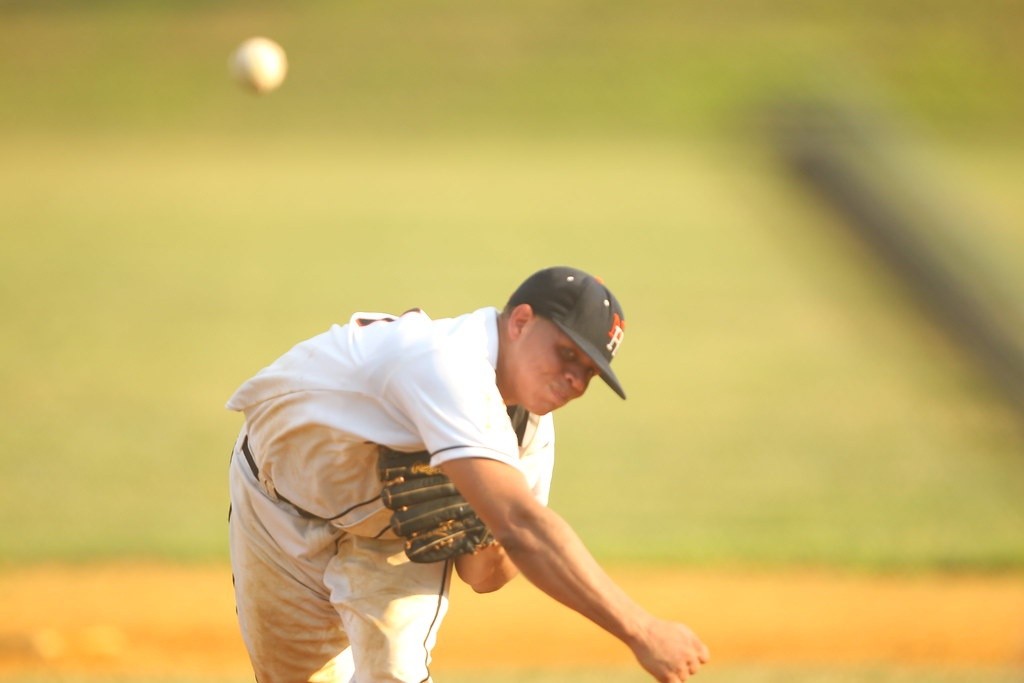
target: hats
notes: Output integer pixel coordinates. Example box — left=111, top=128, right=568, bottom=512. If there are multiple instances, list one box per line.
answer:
left=510, top=266, right=627, bottom=401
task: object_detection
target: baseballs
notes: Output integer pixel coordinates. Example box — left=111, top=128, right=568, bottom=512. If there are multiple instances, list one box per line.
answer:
left=230, top=32, right=292, bottom=98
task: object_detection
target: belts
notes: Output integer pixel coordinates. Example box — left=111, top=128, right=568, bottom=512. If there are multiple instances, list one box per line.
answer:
left=242, top=438, right=318, bottom=518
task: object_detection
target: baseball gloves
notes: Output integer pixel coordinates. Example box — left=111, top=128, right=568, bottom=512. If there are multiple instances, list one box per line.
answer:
left=374, top=446, right=491, bottom=568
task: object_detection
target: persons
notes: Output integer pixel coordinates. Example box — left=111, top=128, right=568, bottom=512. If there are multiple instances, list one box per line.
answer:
left=228, top=267, right=707, bottom=683
